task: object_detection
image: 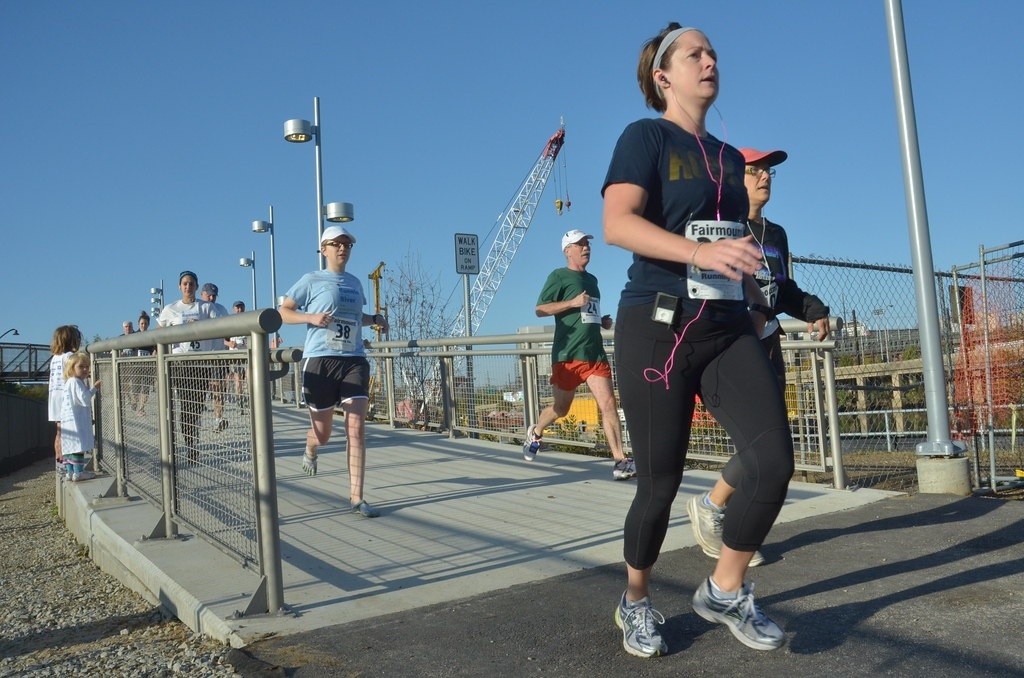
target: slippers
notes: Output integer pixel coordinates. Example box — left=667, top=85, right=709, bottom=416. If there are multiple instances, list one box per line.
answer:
left=302, top=453, right=318, bottom=476
left=351, top=500, right=379, bottom=517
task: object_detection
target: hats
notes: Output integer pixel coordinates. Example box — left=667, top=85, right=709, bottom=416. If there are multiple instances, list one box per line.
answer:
left=561, top=230, right=593, bottom=252
left=321, top=226, right=356, bottom=244
left=233, top=300, right=244, bottom=307
left=203, top=283, right=219, bottom=296
left=738, top=147, right=787, bottom=167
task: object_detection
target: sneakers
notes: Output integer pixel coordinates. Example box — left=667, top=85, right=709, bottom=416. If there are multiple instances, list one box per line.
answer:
left=747, top=550, right=765, bottom=567
left=523, top=425, right=542, bottom=461
left=613, top=458, right=636, bottom=479
left=685, top=491, right=724, bottom=559
left=691, top=576, right=786, bottom=652
left=614, top=588, right=669, bottom=658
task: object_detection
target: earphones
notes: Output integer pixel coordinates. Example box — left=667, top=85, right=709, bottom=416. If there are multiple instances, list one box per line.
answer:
left=661, top=76, right=670, bottom=85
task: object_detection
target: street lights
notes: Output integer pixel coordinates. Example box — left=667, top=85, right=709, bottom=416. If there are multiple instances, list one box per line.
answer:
left=239, top=251, right=257, bottom=312
left=879, top=304, right=893, bottom=361
left=151, top=278, right=164, bottom=316
left=283, top=97, right=355, bottom=270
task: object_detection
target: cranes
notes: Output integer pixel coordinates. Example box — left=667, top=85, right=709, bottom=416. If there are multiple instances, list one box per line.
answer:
left=397, top=116, right=573, bottom=423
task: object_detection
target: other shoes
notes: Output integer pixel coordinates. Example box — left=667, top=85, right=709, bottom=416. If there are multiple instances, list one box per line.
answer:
left=188, top=450, right=199, bottom=464
left=56, top=461, right=67, bottom=474
left=72, top=471, right=96, bottom=482
left=65, top=473, right=74, bottom=481
left=130, top=403, right=136, bottom=410
left=212, top=420, right=229, bottom=433
left=137, top=409, right=145, bottom=415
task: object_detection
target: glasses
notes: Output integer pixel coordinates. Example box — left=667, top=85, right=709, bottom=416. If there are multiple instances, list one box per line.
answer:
left=323, top=241, right=353, bottom=249
left=744, top=166, right=776, bottom=180
left=567, top=240, right=589, bottom=246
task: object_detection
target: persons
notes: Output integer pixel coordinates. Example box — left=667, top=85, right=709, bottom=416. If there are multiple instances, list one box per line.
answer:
left=522, top=229, right=637, bottom=487
left=594, top=22, right=795, bottom=659
left=224, top=301, right=247, bottom=415
left=200, top=283, right=230, bottom=433
left=690, top=149, right=835, bottom=569
left=156, top=271, right=221, bottom=467
left=279, top=226, right=390, bottom=517
left=132, top=310, right=157, bottom=417
left=119, top=320, right=136, bottom=412
left=47, top=324, right=83, bottom=475
left=60, top=352, right=102, bottom=482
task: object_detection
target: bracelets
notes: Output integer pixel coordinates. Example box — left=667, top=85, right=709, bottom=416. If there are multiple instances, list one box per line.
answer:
left=373, top=314, right=383, bottom=324
left=691, top=241, right=703, bottom=275
